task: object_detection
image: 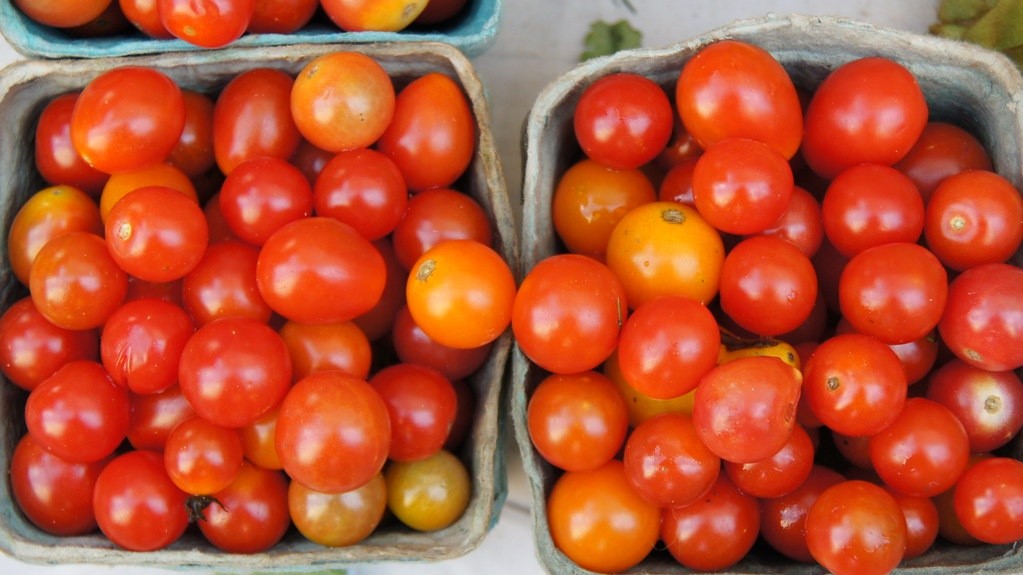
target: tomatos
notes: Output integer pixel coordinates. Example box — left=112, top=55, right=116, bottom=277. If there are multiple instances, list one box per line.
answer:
left=0, top=0, right=1023, bottom=575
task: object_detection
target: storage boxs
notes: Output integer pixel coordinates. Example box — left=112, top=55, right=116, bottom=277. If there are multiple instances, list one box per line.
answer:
left=1, top=0, right=1023, bottom=575
